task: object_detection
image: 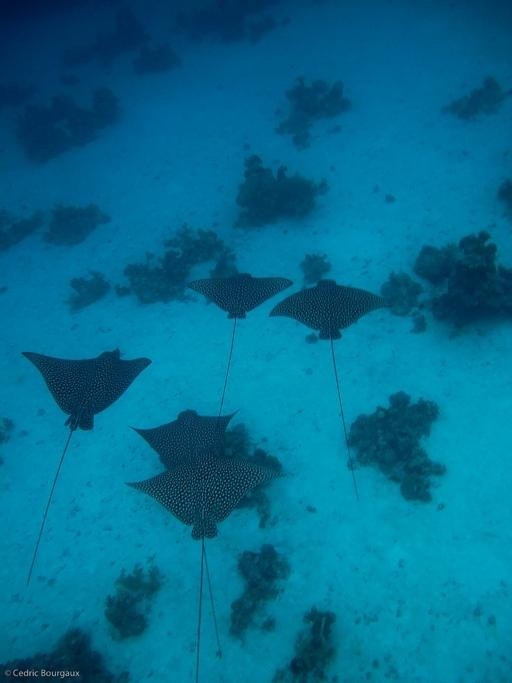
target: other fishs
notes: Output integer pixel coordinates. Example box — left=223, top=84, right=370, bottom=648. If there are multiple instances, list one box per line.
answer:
left=268, top=279, right=390, bottom=498
left=186, top=272, right=294, bottom=430
left=20, top=347, right=152, bottom=587
left=130, top=409, right=241, bottom=644
